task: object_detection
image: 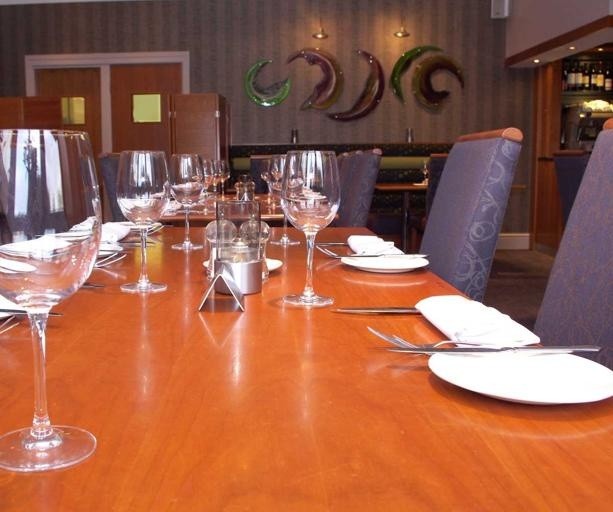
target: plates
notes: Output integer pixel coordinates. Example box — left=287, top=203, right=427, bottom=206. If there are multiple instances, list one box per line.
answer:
left=341, top=256, right=431, bottom=273
left=427, top=351, right=613, bottom=405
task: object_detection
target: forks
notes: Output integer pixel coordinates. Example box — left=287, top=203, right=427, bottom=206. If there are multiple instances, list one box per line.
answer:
left=365, top=326, right=582, bottom=356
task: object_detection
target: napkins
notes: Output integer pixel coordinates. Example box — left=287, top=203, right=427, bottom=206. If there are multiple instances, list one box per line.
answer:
left=0, top=256, right=38, bottom=316
left=0, top=235, right=74, bottom=258
left=344, top=232, right=406, bottom=255
left=164, top=200, right=182, bottom=217
left=71, top=214, right=132, bottom=242
left=413, top=292, right=542, bottom=349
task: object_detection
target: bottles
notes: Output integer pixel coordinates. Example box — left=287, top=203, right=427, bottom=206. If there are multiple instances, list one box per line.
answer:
left=563, top=58, right=613, bottom=93
left=234, top=173, right=256, bottom=229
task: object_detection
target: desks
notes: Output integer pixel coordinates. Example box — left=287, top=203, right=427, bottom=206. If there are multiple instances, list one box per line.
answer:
left=0, top=194, right=613, bottom=510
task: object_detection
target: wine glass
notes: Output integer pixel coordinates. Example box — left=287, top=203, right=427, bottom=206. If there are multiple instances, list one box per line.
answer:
left=267, top=151, right=342, bottom=308
left=171, top=150, right=232, bottom=251
left=1, top=125, right=104, bottom=471
left=117, top=148, right=171, bottom=294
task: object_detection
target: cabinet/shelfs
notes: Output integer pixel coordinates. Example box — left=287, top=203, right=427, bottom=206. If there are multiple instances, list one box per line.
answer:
left=1, top=92, right=226, bottom=222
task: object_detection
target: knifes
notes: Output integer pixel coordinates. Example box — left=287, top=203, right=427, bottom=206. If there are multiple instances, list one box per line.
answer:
left=384, top=343, right=607, bottom=352
left=330, top=305, right=422, bottom=316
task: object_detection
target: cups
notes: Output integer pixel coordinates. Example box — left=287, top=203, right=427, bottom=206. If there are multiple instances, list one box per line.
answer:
left=214, top=200, right=263, bottom=295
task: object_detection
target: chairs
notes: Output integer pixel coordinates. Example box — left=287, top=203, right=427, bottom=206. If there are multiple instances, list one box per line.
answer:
left=325, top=148, right=382, bottom=227
left=411, top=126, right=523, bottom=305
left=497, top=117, right=613, bottom=373
left=82, top=151, right=164, bottom=222
left=1, top=140, right=75, bottom=244
